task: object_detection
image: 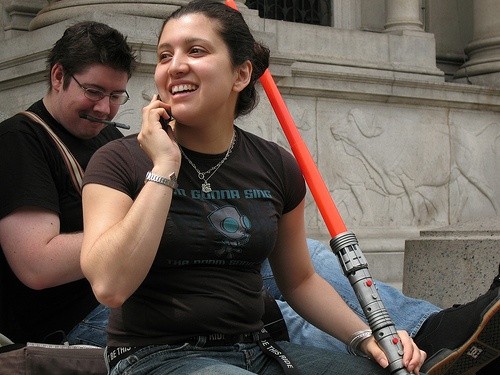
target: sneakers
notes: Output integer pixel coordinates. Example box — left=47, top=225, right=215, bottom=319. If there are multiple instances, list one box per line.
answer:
left=413, top=274, right=500, bottom=375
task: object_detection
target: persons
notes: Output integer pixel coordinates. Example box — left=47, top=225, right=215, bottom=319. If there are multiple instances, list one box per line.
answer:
left=0, top=21, right=500, bottom=375
left=80, top=0, right=427, bottom=375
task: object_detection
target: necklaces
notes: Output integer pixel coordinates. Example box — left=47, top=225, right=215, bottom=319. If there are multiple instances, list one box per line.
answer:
left=176, top=130, right=237, bottom=193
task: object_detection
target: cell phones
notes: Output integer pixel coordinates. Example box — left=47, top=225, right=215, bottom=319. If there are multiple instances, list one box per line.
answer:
left=157, top=95, right=171, bottom=132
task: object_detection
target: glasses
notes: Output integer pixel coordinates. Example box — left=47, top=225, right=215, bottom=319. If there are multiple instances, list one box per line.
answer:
left=70, top=73, right=129, bottom=106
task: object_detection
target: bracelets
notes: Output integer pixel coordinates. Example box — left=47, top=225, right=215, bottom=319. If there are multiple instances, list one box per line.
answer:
left=145, top=171, right=179, bottom=190
left=345, top=329, right=374, bottom=360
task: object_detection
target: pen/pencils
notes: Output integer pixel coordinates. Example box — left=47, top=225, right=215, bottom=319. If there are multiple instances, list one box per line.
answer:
left=79, top=114, right=130, bottom=130
